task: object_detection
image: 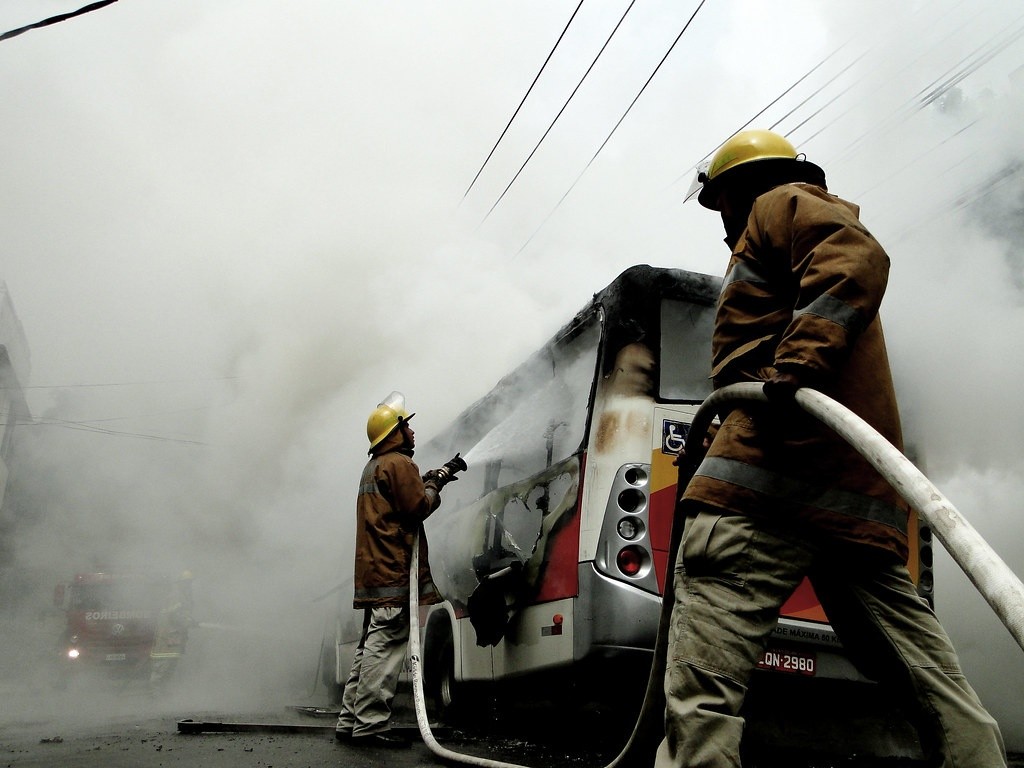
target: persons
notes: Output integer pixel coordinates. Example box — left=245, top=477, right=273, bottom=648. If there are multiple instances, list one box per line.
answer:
left=333, top=406, right=460, bottom=752
left=649, top=132, right=1006, bottom=768
left=147, top=567, right=197, bottom=704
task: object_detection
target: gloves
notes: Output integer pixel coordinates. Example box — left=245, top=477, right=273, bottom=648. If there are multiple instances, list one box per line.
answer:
left=423, top=469, right=458, bottom=482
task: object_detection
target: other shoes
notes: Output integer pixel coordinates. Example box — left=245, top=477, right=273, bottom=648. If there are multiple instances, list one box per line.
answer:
left=336, top=731, right=413, bottom=750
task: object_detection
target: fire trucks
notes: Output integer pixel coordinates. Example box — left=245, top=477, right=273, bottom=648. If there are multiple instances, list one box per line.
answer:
left=53, top=557, right=189, bottom=683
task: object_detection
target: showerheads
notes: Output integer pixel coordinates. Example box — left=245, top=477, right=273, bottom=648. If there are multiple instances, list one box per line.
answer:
left=434, top=453, right=467, bottom=488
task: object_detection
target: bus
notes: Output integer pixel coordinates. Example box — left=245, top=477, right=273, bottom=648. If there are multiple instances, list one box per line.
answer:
left=321, top=263, right=936, bottom=731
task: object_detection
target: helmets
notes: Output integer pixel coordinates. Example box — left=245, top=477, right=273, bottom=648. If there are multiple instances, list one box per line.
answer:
left=698, top=130, right=827, bottom=211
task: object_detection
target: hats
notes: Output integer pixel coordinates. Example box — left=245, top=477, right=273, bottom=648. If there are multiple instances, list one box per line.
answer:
left=364, top=390, right=416, bottom=455
